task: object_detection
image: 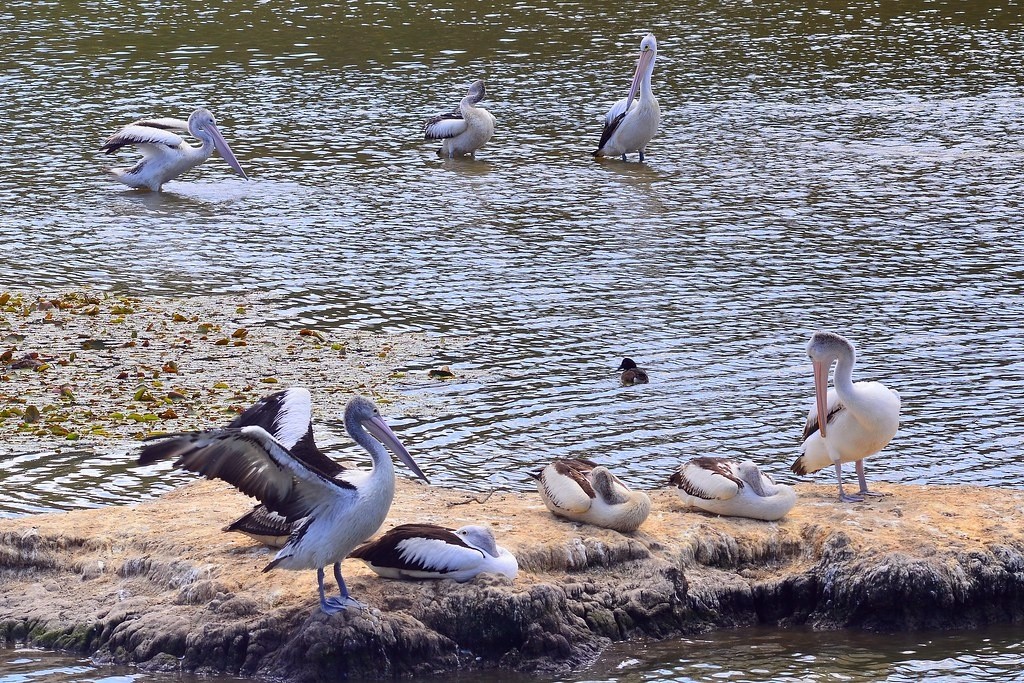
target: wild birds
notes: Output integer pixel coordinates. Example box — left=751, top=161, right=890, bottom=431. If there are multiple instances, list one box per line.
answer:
left=421, top=80, right=497, bottom=159
left=98, top=108, right=249, bottom=192
left=789, top=331, right=900, bottom=504
left=587, top=32, right=660, bottom=161
left=666, top=457, right=796, bottom=521
left=134, top=386, right=519, bottom=616
left=614, top=358, right=649, bottom=387
left=523, top=457, right=651, bottom=534
